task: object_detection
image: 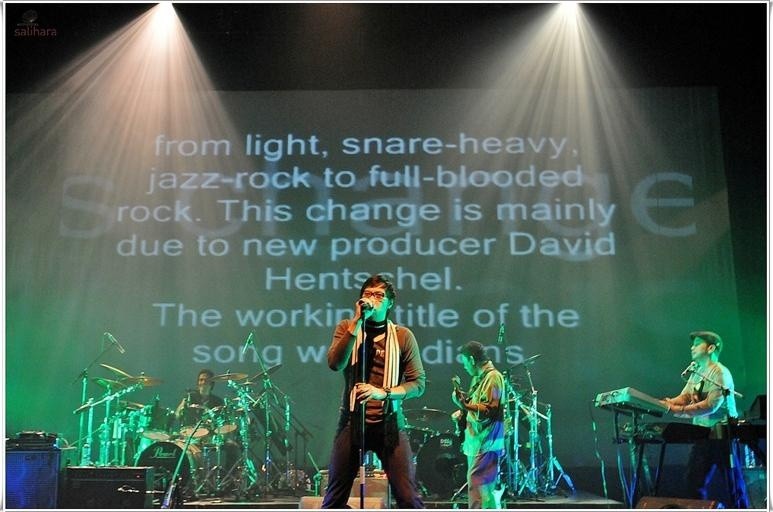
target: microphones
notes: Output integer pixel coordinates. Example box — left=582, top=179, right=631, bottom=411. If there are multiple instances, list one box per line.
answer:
left=103, top=332, right=126, bottom=355
left=242, top=329, right=256, bottom=355
left=185, top=387, right=192, bottom=406
left=498, top=320, right=505, bottom=343
left=363, top=302, right=373, bottom=311
left=681, top=361, right=696, bottom=377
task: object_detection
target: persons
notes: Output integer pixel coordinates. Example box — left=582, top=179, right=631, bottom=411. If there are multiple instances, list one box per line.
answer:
left=452, top=341, right=506, bottom=509
left=661, top=331, right=739, bottom=496
left=321, top=276, right=427, bottom=509
left=176, top=370, right=225, bottom=422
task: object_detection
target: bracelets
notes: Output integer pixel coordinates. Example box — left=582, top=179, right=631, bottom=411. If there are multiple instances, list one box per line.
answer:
left=381, top=388, right=392, bottom=402
left=680, top=406, right=684, bottom=414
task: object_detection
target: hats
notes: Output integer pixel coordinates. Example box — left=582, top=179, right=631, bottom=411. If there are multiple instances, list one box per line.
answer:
left=687, top=330, right=725, bottom=353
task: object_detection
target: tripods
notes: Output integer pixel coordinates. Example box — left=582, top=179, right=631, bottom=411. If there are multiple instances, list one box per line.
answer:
left=195, top=432, right=238, bottom=496
left=222, top=418, right=259, bottom=498
left=275, top=402, right=300, bottom=498
left=500, top=381, right=514, bottom=498
left=258, top=399, right=285, bottom=498
left=505, top=400, right=527, bottom=498
left=514, top=397, right=553, bottom=499
left=300, top=439, right=315, bottom=497
left=539, top=405, right=576, bottom=495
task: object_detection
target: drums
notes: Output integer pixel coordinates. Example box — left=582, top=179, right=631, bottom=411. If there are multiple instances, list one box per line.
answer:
left=138, top=404, right=175, bottom=441
left=134, top=440, right=204, bottom=502
left=417, top=434, right=467, bottom=498
left=193, top=440, right=242, bottom=479
left=515, top=441, right=538, bottom=470
left=404, top=425, right=433, bottom=453
left=117, top=400, right=145, bottom=431
left=178, top=404, right=210, bottom=438
left=211, top=403, right=238, bottom=434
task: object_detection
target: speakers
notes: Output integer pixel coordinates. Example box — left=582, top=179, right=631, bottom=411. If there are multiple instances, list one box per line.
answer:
left=60, top=466, right=154, bottom=509
left=61, top=448, right=78, bottom=466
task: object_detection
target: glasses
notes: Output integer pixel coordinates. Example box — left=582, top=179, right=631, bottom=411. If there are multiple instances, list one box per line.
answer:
left=362, top=289, right=392, bottom=300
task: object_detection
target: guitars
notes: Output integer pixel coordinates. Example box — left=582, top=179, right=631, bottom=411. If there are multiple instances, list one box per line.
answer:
left=451, top=375, right=468, bottom=443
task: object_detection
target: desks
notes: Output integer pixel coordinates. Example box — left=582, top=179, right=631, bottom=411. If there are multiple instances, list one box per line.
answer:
left=613, top=437, right=709, bottom=509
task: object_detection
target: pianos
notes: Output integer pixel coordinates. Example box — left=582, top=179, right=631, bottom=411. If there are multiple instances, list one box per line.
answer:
left=593, top=387, right=671, bottom=414
left=618, top=421, right=711, bottom=444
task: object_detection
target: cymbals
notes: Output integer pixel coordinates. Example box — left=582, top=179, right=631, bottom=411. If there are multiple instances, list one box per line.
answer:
left=99, top=362, right=134, bottom=379
left=91, top=376, right=124, bottom=391
left=232, top=396, right=261, bottom=401
left=236, top=407, right=252, bottom=412
left=208, top=373, right=248, bottom=383
left=402, top=406, right=450, bottom=422
left=508, top=353, right=543, bottom=369
left=119, top=376, right=165, bottom=388
left=507, top=389, right=529, bottom=404
left=248, top=364, right=282, bottom=382
